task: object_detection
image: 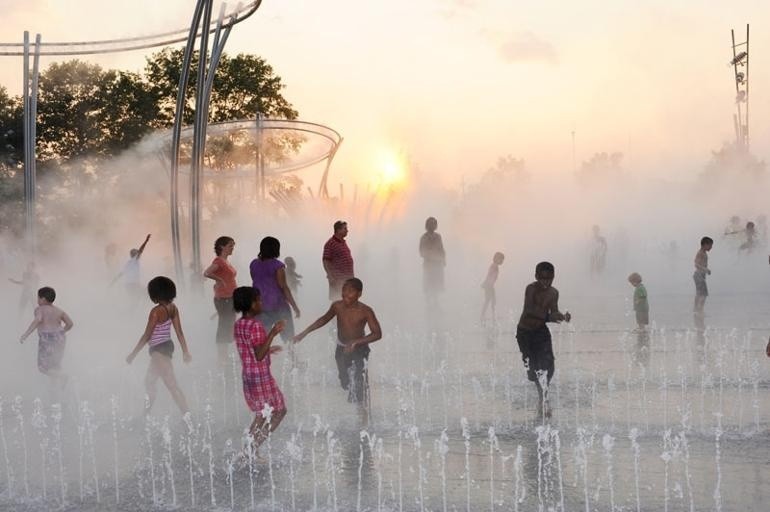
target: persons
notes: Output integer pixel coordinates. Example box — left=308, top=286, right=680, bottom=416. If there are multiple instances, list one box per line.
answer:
left=323, top=219, right=357, bottom=333
left=628, top=271, right=652, bottom=329
left=725, top=221, right=760, bottom=256
left=417, top=216, right=448, bottom=297
left=201, top=236, right=237, bottom=362
left=515, top=261, right=571, bottom=420
left=479, top=251, right=506, bottom=321
left=126, top=275, right=194, bottom=419
left=725, top=217, right=743, bottom=240
left=293, top=276, right=383, bottom=427
left=19, top=286, right=75, bottom=376
left=123, top=232, right=152, bottom=299
left=584, top=224, right=609, bottom=282
left=226, top=286, right=289, bottom=466
left=693, top=235, right=715, bottom=315
left=284, top=255, right=301, bottom=295
left=248, top=234, right=302, bottom=352
